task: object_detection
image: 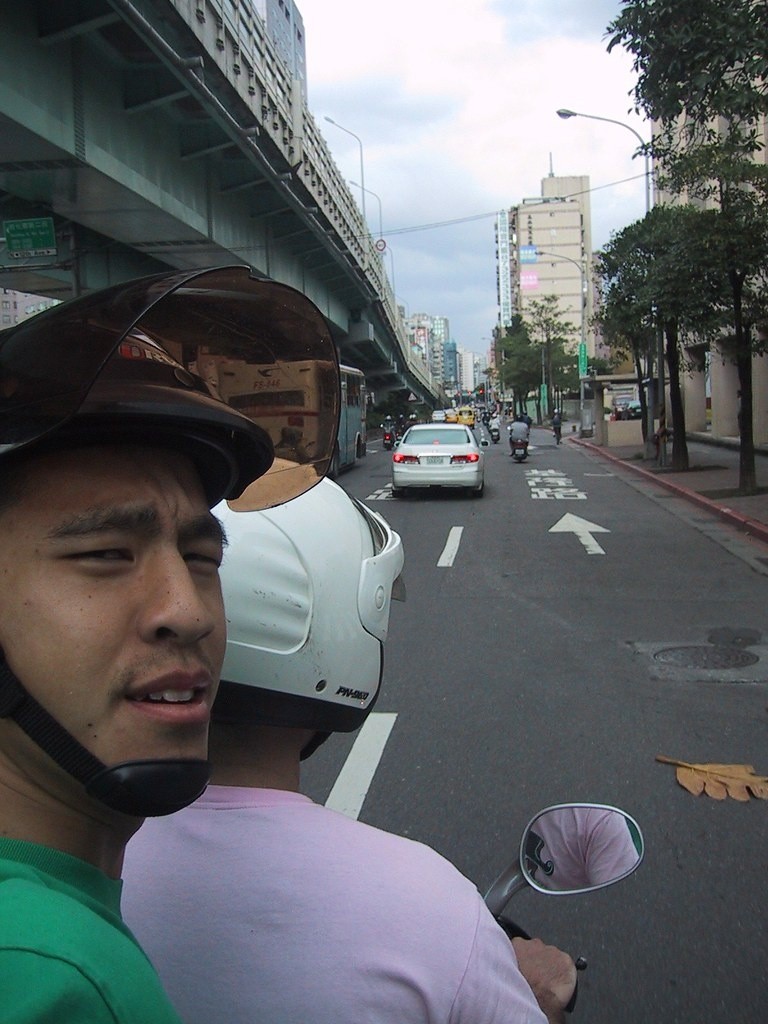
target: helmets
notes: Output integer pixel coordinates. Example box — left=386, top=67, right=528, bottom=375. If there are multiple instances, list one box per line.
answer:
left=210, top=456, right=405, bottom=732
left=0, top=318, right=274, bottom=511
left=385, top=415, right=392, bottom=420
left=491, top=413, right=497, bottom=418
left=410, top=414, right=413, bottom=419
left=516, top=414, right=524, bottom=421
left=524, top=411, right=527, bottom=415
left=399, top=414, right=404, bottom=419
left=489, top=411, right=493, bottom=415
left=413, top=414, right=416, bottom=419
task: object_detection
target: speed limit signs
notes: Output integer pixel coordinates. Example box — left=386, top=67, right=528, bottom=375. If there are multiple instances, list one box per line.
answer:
left=376, top=239, right=387, bottom=253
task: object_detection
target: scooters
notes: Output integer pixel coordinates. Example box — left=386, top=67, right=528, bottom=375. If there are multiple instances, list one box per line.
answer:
left=490, top=428, right=500, bottom=444
left=380, top=424, right=397, bottom=451
left=507, top=426, right=530, bottom=464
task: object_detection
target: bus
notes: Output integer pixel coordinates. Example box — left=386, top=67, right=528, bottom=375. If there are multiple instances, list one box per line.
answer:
left=218, top=357, right=373, bottom=481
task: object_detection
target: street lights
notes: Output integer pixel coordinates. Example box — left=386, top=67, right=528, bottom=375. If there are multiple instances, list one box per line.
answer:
left=557, top=108, right=669, bottom=469
left=535, top=250, right=586, bottom=431
left=323, top=116, right=365, bottom=221
left=350, top=182, right=383, bottom=257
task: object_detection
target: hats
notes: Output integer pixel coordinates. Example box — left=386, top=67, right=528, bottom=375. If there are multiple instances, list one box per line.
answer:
left=554, top=409, right=558, bottom=414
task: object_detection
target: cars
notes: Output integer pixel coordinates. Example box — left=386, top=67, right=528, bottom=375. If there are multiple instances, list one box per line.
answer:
left=392, top=422, right=489, bottom=497
left=445, top=412, right=457, bottom=423
left=431, top=411, right=447, bottom=422
left=610, top=393, right=642, bottom=421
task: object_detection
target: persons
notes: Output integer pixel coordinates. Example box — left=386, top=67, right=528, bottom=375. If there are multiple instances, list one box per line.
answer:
left=549, top=409, right=563, bottom=441
left=120, top=457, right=579, bottom=1024
left=0, top=264, right=342, bottom=1024
left=382, top=413, right=421, bottom=444
left=476, top=409, right=501, bottom=440
left=504, top=407, right=533, bottom=457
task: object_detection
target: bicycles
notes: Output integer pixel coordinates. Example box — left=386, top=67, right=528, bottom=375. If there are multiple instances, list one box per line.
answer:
left=554, top=426, right=560, bottom=446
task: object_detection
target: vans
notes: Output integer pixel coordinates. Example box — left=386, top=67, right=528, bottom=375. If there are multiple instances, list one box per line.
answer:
left=456, top=406, right=477, bottom=429
